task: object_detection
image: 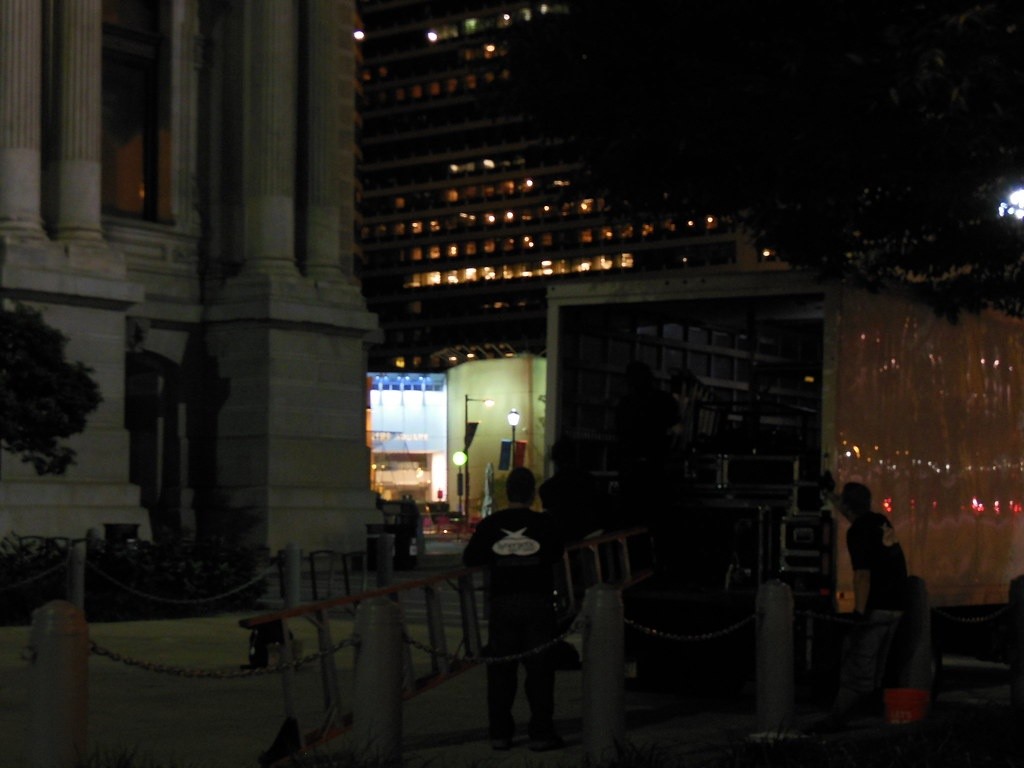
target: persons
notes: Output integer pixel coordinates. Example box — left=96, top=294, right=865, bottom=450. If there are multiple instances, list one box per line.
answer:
left=811, top=483, right=908, bottom=734
left=462, top=467, right=566, bottom=751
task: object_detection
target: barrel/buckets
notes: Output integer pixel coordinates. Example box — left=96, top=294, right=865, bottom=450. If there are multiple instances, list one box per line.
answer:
left=883, top=688, right=928, bottom=725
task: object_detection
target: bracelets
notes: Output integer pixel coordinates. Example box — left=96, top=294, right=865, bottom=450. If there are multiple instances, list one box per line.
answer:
left=823, top=488, right=829, bottom=494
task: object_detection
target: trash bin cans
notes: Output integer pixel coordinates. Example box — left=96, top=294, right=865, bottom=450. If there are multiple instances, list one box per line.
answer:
left=364, top=523, right=396, bottom=585
left=101, top=521, right=141, bottom=543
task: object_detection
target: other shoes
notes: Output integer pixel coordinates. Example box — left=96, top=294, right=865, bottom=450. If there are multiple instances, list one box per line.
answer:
left=491, top=735, right=513, bottom=749
left=528, top=736, right=563, bottom=751
